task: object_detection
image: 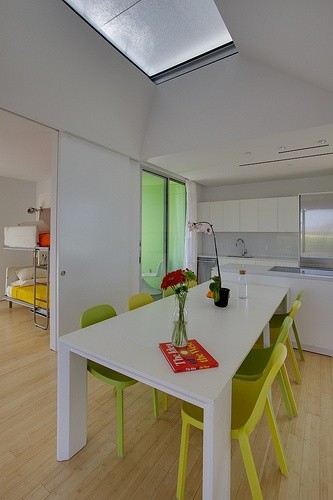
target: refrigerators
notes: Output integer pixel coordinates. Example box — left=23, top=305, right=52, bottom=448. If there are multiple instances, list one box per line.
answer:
left=298, top=193, right=333, bottom=276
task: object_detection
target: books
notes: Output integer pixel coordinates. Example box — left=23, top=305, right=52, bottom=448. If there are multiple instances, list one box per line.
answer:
left=159, top=340, right=219, bottom=374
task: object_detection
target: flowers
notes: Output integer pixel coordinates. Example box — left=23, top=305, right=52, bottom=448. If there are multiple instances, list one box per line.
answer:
left=160, top=268, right=197, bottom=345
left=187, top=220, right=221, bottom=287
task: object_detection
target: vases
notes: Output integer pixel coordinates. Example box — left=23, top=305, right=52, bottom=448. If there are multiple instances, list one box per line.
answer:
left=172, top=299, right=189, bottom=348
left=214, top=288, right=230, bottom=307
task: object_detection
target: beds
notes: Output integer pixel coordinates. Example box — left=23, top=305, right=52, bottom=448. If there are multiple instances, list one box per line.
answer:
left=2, top=226, right=49, bottom=330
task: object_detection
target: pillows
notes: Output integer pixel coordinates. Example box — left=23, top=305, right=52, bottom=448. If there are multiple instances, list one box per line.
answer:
left=9, top=278, right=47, bottom=288
left=15, top=268, right=47, bottom=280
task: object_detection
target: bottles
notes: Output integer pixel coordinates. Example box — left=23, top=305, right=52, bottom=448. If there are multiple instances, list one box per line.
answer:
left=238, top=269, right=247, bottom=299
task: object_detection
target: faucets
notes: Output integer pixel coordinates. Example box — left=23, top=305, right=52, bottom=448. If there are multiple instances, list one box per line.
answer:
left=235, top=238, right=248, bottom=256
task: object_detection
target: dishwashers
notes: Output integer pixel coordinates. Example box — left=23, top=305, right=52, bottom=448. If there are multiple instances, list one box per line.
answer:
left=197, top=257, right=217, bottom=285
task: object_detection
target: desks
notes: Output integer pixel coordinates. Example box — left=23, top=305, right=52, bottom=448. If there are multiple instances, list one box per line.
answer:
left=56, top=279, right=291, bottom=500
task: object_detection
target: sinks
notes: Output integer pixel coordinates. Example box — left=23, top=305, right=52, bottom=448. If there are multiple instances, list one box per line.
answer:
left=226, top=255, right=253, bottom=258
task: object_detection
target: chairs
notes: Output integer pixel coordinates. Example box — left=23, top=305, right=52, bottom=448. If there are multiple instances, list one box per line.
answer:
left=163, top=279, right=197, bottom=297
left=236, top=290, right=305, bottom=420
left=129, top=292, right=169, bottom=412
left=80, top=303, right=160, bottom=457
left=176, top=343, right=288, bottom=500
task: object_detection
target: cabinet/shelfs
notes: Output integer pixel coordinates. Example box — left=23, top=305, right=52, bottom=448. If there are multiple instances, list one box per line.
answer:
left=196, top=195, right=298, bottom=233
